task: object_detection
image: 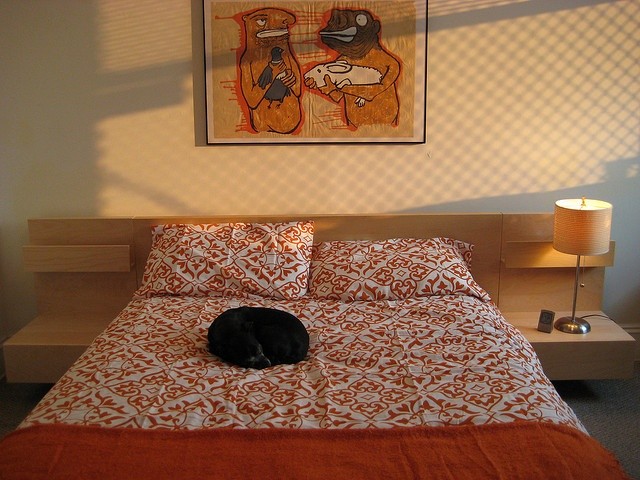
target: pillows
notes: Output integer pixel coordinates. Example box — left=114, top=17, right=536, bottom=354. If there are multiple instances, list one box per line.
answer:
left=309, top=238, right=492, bottom=302
left=134, top=219, right=315, bottom=299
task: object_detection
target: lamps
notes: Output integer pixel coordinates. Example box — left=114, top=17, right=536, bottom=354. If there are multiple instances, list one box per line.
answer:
left=552, top=198, right=612, bottom=333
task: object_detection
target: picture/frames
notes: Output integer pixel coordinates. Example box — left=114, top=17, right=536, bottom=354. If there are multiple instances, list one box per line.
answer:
left=202, top=0, right=429, bottom=145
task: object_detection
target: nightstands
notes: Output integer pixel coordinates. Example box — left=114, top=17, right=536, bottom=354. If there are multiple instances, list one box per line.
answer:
left=3, top=216, right=138, bottom=384
left=499, top=211, right=637, bottom=381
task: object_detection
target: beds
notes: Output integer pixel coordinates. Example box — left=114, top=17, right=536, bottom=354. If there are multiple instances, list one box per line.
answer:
left=1, top=213, right=633, bottom=479
